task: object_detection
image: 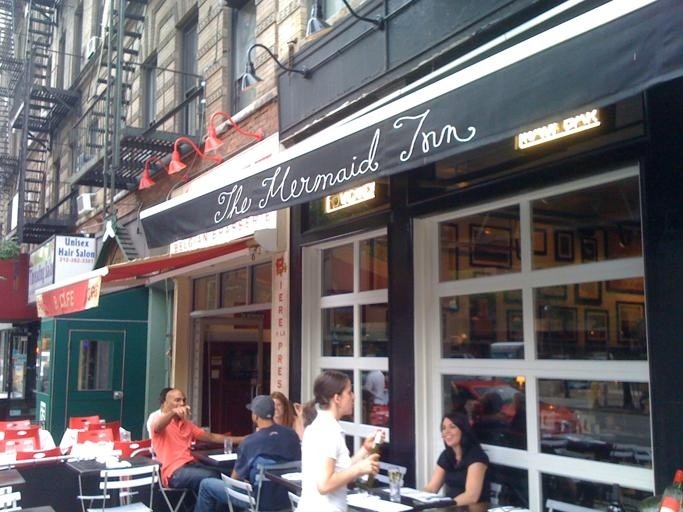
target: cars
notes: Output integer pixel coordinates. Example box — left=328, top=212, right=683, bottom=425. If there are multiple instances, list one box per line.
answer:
left=439, top=344, right=613, bottom=456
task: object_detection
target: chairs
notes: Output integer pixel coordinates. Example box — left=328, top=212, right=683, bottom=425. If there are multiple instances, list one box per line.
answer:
left=539, top=432, right=653, bottom=496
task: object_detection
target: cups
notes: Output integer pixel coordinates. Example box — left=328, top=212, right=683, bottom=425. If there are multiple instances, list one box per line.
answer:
left=387, top=467, right=404, bottom=500
left=222, top=437, right=235, bottom=455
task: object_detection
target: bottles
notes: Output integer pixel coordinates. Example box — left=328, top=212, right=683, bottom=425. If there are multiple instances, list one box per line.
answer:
left=608, top=481, right=624, bottom=511
left=357, top=424, right=384, bottom=490
left=661, top=468, right=682, bottom=512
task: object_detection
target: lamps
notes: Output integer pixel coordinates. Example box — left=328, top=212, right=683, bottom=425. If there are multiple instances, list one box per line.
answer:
left=139, top=111, right=264, bottom=189
left=242, top=0, right=387, bottom=87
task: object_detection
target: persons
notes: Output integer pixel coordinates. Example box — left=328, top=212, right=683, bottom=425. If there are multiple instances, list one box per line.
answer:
left=498, top=392, right=525, bottom=432
left=193, top=395, right=300, bottom=511
left=146, top=386, right=174, bottom=440
left=295, top=368, right=386, bottom=512
left=150, top=390, right=248, bottom=491
left=423, top=412, right=490, bottom=506
left=270, top=392, right=304, bottom=439
left=464, top=391, right=517, bottom=488
left=363, top=368, right=388, bottom=407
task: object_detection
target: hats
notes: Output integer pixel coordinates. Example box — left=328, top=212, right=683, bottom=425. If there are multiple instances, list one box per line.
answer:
left=246, top=394, right=275, bottom=420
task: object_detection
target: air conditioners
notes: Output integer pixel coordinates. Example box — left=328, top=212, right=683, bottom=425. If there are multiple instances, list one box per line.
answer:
left=86, top=36, right=98, bottom=59
left=76, top=193, right=91, bottom=216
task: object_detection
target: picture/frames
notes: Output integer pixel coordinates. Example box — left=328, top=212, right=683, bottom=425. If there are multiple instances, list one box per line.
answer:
left=467, top=221, right=645, bottom=345
left=324, top=288, right=367, bottom=337
left=368, top=222, right=459, bottom=313
